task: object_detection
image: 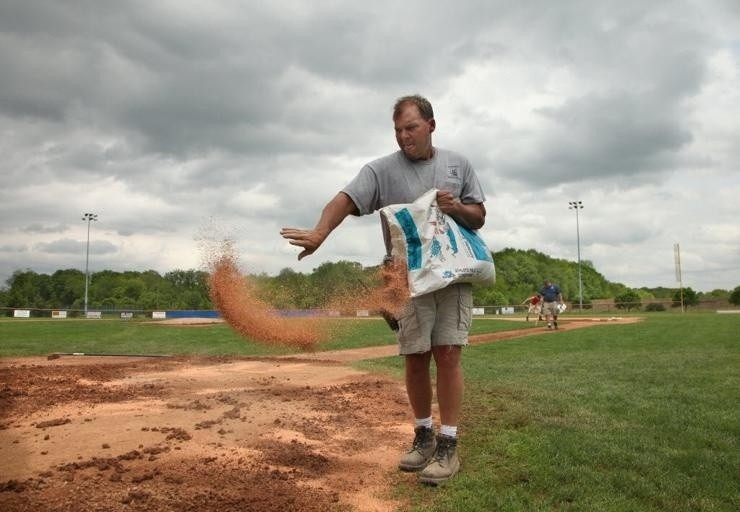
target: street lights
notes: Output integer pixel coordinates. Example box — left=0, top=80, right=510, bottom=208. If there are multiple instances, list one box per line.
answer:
left=567, top=200, right=584, bottom=313
left=80, top=212, right=98, bottom=316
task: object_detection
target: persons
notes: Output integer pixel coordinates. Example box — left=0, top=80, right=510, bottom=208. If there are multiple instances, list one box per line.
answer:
left=522, top=295, right=544, bottom=322
left=540, top=280, right=564, bottom=330
left=280, top=95, right=485, bottom=485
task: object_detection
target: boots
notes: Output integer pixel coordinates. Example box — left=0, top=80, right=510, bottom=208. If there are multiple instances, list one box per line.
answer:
left=399, top=426, right=437, bottom=468
left=421, top=433, right=459, bottom=483
